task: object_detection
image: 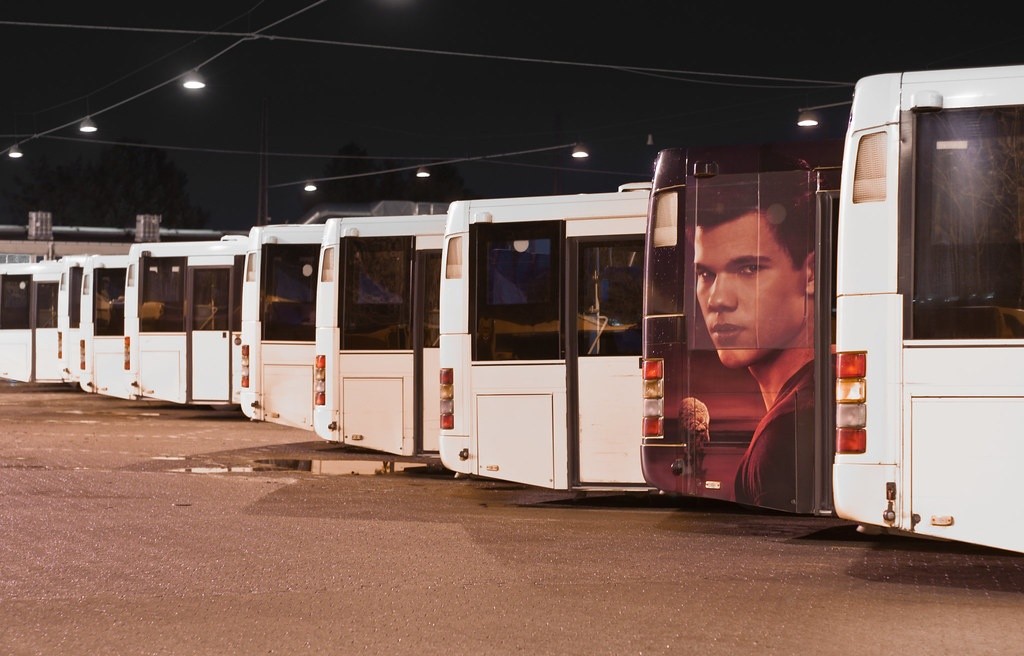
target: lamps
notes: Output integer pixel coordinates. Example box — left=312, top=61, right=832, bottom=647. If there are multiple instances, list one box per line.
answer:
left=416, top=142, right=431, bottom=177
left=79, top=95, right=97, bottom=133
left=304, top=156, right=317, bottom=191
left=8, top=115, right=24, bottom=157
left=797, top=88, right=819, bottom=127
left=571, top=122, right=590, bottom=158
left=182, top=40, right=205, bottom=89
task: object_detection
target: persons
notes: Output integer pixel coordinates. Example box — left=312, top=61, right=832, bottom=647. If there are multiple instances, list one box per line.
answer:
left=694, top=171, right=834, bottom=510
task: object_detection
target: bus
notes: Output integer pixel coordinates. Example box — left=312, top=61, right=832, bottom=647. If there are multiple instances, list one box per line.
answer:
left=314, top=214, right=451, bottom=458
left=640, top=134, right=846, bottom=517
left=439, top=180, right=657, bottom=494
left=832, top=64, right=1024, bottom=553
left=58, top=253, right=89, bottom=383
left=0, top=260, right=80, bottom=392
left=124, top=234, right=250, bottom=406
left=239, top=223, right=333, bottom=431
left=79, top=254, right=139, bottom=401
